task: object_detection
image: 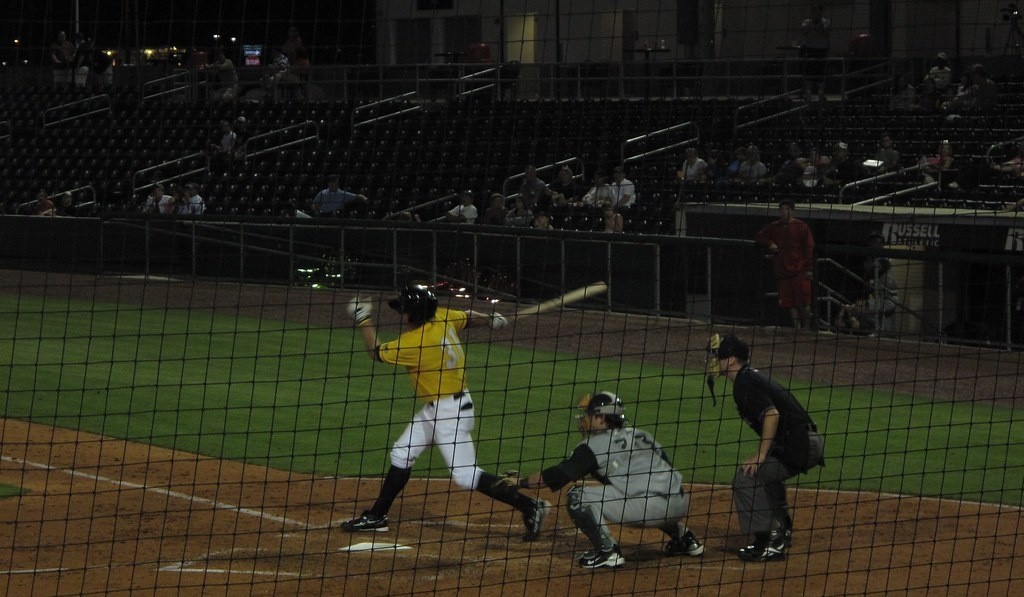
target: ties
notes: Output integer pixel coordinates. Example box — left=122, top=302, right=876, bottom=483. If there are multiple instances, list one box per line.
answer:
left=154, top=202, right=159, bottom=214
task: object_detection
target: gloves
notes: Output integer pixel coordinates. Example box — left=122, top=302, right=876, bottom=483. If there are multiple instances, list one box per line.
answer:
left=489, top=312, right=509, bottom=330
left=344, top=296, right=375, bottom=325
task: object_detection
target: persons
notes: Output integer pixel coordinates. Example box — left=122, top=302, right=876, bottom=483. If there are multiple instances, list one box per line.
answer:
left=847, top=33, right=882, bottom=66
left=192, top=48, right=237, bottom=99
left=310, top=173, right=367, bottom=213
left=141, top=180, right=204, bottom=216
left=263, top=28, right=310, bottom=101
left=916, top=50, right=992, bottom=112
left=759, top=199, right=816, bottom=328
left=703, top=333, right=824, bottom=561
left=211, top=114, right=254, bottom=155
left=341, top=284, right=551, bottom=541
left=47, top=27, right=113, bottom=91
left=677, top=141, right=882, bottom=207
left=858, top=258, right=898, bottom=333
left=797, top=4, right=832, bottom=95
left=923, top=137, right=1024, bottom=212
left=446, top=164, right=635, bottom=232
left=888, top=73, right=916, bottom=111
left=874, top=134, right=901, bottom=173
left=503, top=391, right=706, bottom=566
left=35, top=189, right=57, bottom=217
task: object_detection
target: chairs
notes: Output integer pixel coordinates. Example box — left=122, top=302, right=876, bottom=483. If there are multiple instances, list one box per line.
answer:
left=0, top=84, right=1024, bottom=226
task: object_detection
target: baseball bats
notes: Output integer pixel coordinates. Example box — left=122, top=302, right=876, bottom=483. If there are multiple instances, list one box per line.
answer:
left=506, top=280, right=608, bottom=322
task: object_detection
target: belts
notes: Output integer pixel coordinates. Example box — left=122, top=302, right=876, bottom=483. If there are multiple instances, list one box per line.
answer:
left=807, top=422, right=818, bottom=430
left=427, top=389, right=465, bottom=408
left=662, top=486, right=684, bottom=500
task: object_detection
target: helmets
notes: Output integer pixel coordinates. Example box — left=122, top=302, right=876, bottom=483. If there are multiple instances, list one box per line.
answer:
left=387, top=283, right=438, bottom=327
left=576, top=391, right=628, bottom=416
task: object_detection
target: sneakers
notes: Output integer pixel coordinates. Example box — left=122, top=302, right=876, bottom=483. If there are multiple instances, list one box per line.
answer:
left=739, top=541, right=789, bottom=561
left=523, top=498, right=552, bottom=541
left=665, top=534, right=704, bottom=556
left=340, top=511, right=391, bottom=532
left=578, top=543, right=625, bottom=568
left=737, top=538, right=771, bottom=559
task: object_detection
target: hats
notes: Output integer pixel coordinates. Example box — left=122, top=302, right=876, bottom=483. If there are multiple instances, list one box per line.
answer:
left=718, top=336, right=750, bottom=358
left=614, top=165, right=624, bottom=174
left=63, top=190, right=72, bottom=198
